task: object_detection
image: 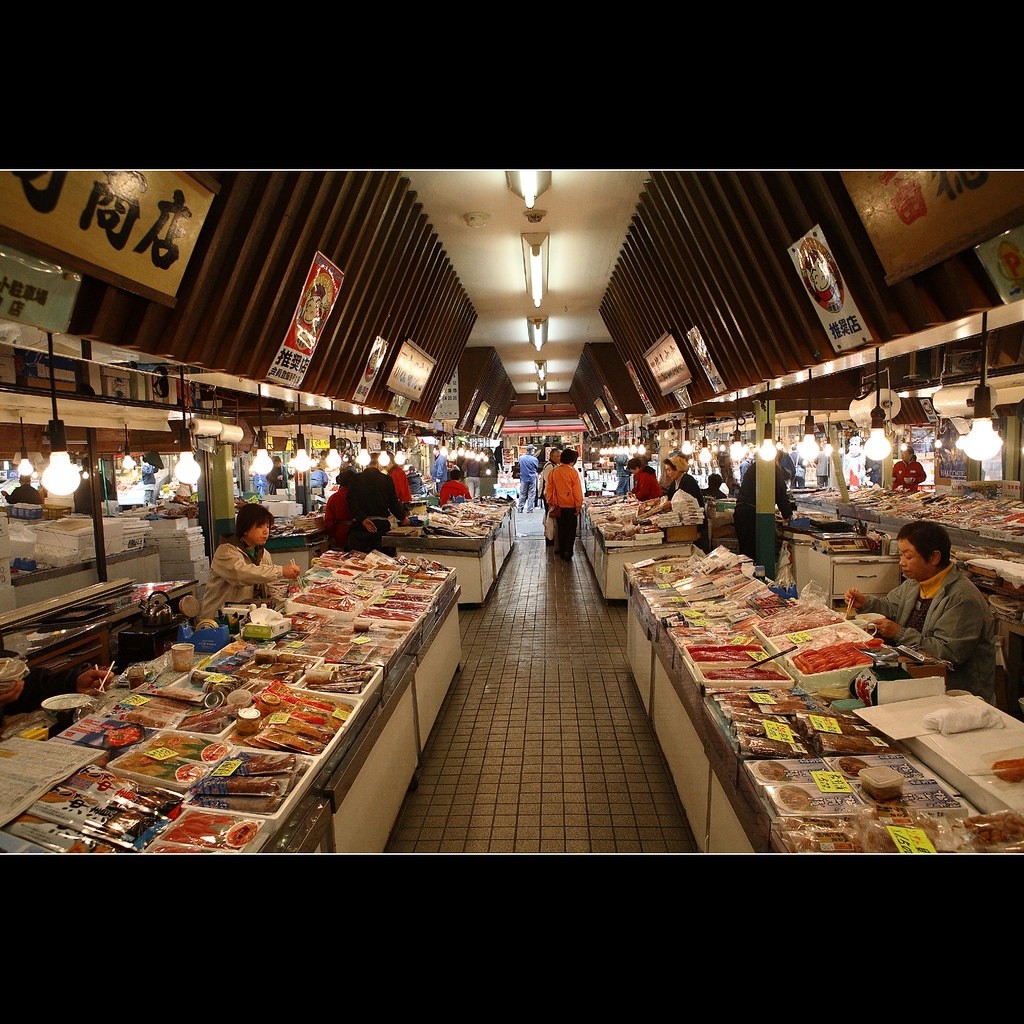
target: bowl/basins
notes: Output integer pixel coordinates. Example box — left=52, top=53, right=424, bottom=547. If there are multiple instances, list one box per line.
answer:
left=305, top=669, right=331, bottom=683
left=855, top=612, right=886, bottom=629
left=41, top=693, right=91, bottom=722
left=254, top=649, right=277, bottom=664
left=754, top=762, right=794, bottom=782
left=772, top=786, right=817, bottom=812
left=267, top=582, right=289, bottom=598
left=858, top=766, right=905, bottom=800
left=201, top=743, right=228, bottom=764
left=175, top=764, right=209, bottom=782
left=226, top=821, right=259, bottom=847
left=832, top=757, right=871, bottom=779
left=354, top=620, right=369, bottom=631
left=0, top=658, right=30, bottom=694
left=103, top=723, right=145, bottom=750
left=227, top=690, right=253, bottom=706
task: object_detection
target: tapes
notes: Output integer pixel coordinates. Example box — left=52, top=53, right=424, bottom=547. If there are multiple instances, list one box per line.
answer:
left=195, top=619, right=218, bottom=631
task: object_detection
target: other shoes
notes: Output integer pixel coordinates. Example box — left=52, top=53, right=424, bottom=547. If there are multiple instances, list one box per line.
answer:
left=527, top=510, right=533, bottom=513
left=560, top=554, right=570, bottom=561
left=518, top=508, right=523, bottom=512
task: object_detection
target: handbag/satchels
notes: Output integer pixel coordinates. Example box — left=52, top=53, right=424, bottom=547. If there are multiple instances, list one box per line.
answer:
left=545, top=507, right=555, bottom=540
left=795, top=465, right=805, bottom=479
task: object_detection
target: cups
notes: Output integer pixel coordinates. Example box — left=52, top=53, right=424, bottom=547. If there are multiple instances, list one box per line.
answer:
left=945, top=689, right=985, bottom=703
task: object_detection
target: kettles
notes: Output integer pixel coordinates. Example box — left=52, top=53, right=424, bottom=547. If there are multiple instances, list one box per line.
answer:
left=139, top=590, right=172, bottom=626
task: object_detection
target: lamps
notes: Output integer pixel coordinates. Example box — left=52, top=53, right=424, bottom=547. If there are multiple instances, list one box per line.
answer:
left=536, top=383, right=547, bottom=396
left=506, top=172, right=553, bottom=209
left=523, top=232, right=551, bottom=307
left=535, top=360, right=548, bottom=380
left=527, top=316, right=550, bottom=350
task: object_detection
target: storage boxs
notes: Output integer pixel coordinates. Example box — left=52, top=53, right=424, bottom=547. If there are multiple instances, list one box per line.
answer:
left=0, top=474, right=515, bottom=854
left=580, top=445, right=1024, bottom=855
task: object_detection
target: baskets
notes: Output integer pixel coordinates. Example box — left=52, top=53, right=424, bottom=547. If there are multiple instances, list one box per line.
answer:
left=6, top=504, right=43, bottom=519
left=41, top=503, right=71, bottom=520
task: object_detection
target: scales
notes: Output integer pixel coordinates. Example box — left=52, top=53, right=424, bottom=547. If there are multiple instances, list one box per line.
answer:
left=810, top=531, right=870, bottom=554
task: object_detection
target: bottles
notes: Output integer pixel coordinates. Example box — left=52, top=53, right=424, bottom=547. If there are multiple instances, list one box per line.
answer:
left=756, top=566, right=765, bottom=581
left=661, top=612, right=689, bottom=628
left=236, top=707, right=260, bottom=735
left=129, top=667, right=144, bottom=689
left=171, top=643, right=194, bottom=671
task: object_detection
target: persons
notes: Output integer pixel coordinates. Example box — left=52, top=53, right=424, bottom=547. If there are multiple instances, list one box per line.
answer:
left=701, top=473, right=727, bottom=500
left=546, top=449, right=583, bottom=560
left=542, top=448, right=561, bottom=546
left=432, top=439, right=504, bottom=506
left=324, top=466, right=358, bottom=552
left=1, top=475, right=42, bottom=505
left=614, top=454, right=631, bottom=494
left=253, top=455, right=423, bottom=514
left=74, top=457, right=112, bottom=513
left=663, top=456, right=709, bottom=555
left=844, top=521, right=996, bottom=702
left=347, top=456, right=410, bottom=558
left=142, top=460, right=159, bottom=505
left=740, top=446, right=806, bottom=489
left=892, top=446, right=926, bottom=492
left=199, top=503, right=300, bottom=620
left=814, top=451, right=882, bottom=487
left=732, top=462, right=793, bottom=562
left=627, top=458, right=663, bottom=501
left=518, top=444, right=539, bottom=513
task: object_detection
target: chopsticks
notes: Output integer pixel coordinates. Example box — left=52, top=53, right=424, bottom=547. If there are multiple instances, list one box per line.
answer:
left=290, top=558, right=304, bottom=590
left=94, top=660, right=116, bottom=693
left=845, top=586, right=858, bottom=617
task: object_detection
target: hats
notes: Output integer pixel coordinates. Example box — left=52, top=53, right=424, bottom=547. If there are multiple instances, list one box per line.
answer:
left=668, top=455, right=689, bottom=472
left=527, top=445, right=537, bottom=451
